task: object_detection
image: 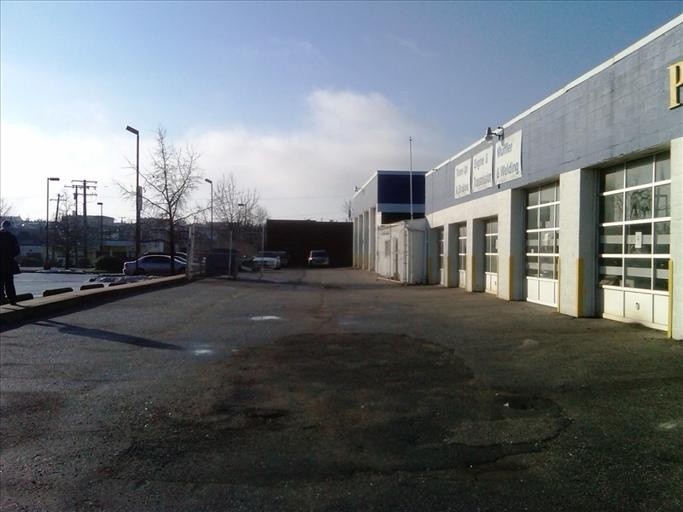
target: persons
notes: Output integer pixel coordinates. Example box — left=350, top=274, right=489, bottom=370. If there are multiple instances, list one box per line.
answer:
left=0, top=219, right=20, bottom=305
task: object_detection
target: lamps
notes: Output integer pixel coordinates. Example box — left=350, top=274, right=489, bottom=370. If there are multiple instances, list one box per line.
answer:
left=485, top=127, right=504, bottom=143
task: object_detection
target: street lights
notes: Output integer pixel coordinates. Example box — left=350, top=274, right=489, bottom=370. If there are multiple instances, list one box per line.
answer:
left=126, top=125, right=139, bottom=272
left=205, top=177, right=214, bottom=238
left=407, top=134, right=416, bottom=222
left=44, top=178, right=60, bottom=270
left=238, top=204, right=247, bottom=229
left=97, top=203, right=103, bottom=258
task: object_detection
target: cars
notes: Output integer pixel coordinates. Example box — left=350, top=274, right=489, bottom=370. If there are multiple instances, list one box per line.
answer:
left=122, top=251, right=189, bottom=276
left=308, top=249, right=331, bottom=267
left=240, top=250, right=290, bottom=272
